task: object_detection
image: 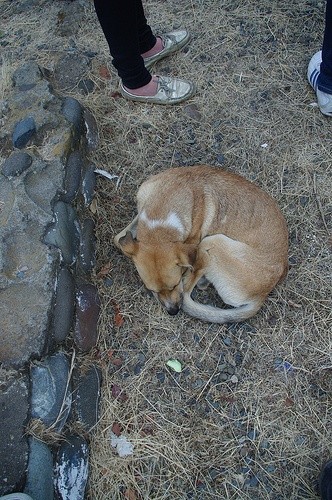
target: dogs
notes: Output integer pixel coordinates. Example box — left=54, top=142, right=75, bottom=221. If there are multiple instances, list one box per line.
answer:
left=113, top=166, right=287, bottom=323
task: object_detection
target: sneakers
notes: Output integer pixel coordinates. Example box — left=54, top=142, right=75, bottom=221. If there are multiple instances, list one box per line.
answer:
left=142, top=29, right=191, bottom=71
left=119, top=74, right=195, bottom=104
left=307, top=51, right=332, bottom=116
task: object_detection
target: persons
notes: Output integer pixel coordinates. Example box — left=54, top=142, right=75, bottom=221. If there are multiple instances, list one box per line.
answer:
left=307, top=0, right=332, bottom=116
left=94, top=0, right=195, bottom=105
